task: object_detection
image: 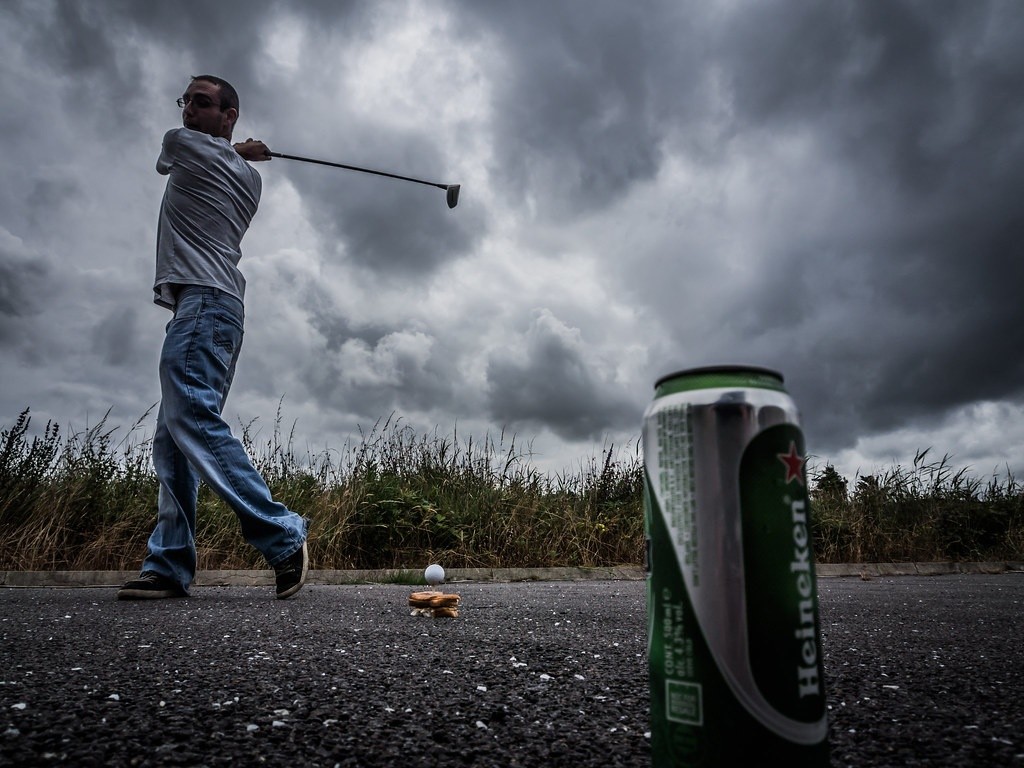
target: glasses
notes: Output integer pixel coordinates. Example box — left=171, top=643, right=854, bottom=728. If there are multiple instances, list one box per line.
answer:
left=176, top=97, right=221, bottom=108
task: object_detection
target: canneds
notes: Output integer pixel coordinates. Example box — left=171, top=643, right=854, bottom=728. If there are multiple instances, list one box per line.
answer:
left=640, top=363, right=829, bottom=768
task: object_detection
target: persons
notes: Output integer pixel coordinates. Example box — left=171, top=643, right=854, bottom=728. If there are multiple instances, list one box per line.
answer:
left=117, top=75, right=310, bottom=600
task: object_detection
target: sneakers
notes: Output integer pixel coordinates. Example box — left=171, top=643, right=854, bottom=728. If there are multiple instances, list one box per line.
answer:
left=273, top=540, right=309, bottom=600
left=118, top=572, right=186, bottom=598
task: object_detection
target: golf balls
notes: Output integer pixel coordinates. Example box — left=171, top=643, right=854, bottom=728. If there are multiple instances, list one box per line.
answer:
left=424, top=564, right=446, bottom=585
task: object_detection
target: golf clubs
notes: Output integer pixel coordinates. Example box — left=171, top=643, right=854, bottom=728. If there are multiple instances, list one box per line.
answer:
left=265, top=150, right=461, bottom=209
